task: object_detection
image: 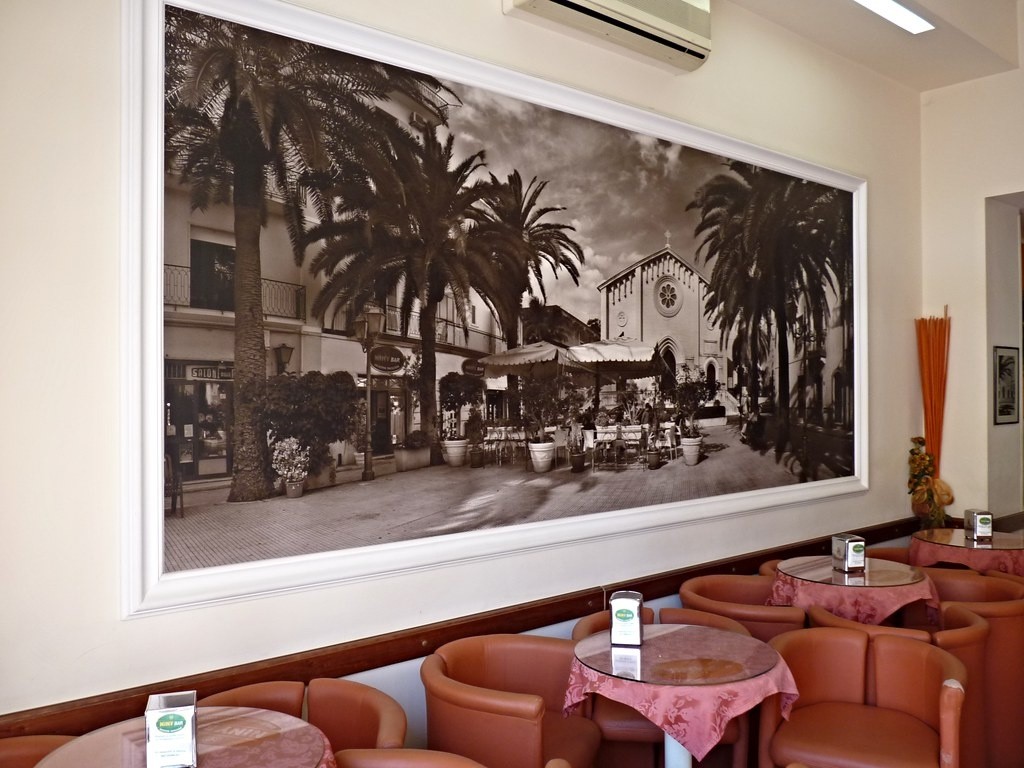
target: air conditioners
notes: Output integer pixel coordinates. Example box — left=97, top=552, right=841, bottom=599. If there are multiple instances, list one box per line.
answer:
left=501, top=0, right=713, bottom=74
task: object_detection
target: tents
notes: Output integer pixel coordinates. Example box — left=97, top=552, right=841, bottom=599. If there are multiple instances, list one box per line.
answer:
left=477, top=340, right=677, bottom=444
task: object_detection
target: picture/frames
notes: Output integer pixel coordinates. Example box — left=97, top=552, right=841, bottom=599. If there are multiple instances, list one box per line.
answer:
left=119, top=0, right=870, bottom=621
left=993, top=345, right=1022, bottom=425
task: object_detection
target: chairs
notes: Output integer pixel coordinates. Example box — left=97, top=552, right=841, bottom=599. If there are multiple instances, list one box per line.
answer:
left=35, top=513, right=1023, bottom=768
